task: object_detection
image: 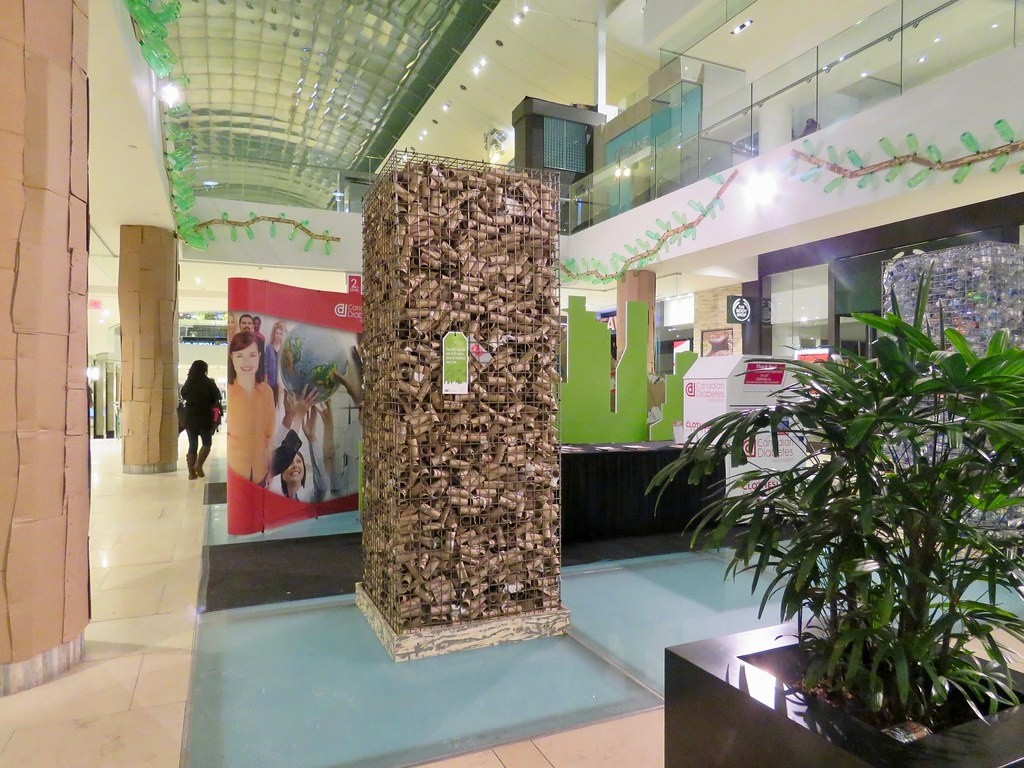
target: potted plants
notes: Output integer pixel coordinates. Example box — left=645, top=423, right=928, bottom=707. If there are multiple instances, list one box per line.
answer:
left=645, top=258, right=1024, bottom=768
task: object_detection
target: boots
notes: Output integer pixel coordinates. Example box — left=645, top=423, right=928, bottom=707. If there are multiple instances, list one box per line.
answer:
left=186, top=453, right=197, bottom=480
left=192, top=445, right=210, bottom=477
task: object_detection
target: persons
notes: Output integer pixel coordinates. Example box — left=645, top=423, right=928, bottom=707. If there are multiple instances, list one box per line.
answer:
left=226, top=312, right=365, bottom=510
left=800, top=118, right=821, bottom=137
left=179, top=360, right=223, bottom=482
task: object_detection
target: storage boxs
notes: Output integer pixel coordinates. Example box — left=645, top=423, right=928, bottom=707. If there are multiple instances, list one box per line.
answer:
left=683, top=354, right=807, bottom=510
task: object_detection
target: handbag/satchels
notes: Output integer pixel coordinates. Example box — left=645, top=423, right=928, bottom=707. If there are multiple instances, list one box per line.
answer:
left=211, top=399, right=223, bottom=427
left=177, top=397, right=187, bottom=427
